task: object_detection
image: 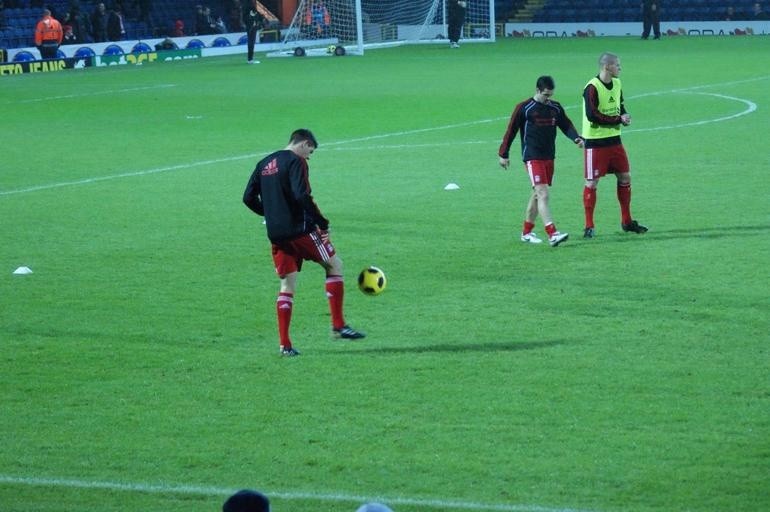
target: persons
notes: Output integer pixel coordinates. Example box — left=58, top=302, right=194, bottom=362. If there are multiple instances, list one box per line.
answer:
left=241, top=128, right=366, bottom=354
left=582, top=53, right=649, bottom=236
left=638, top=0, right=660, bottom=40
left=221, top=487, right=270, bottom=510
left=752, top=3, right=767, bottom=19
left=35, top=0, right=331, bottom=65
left=721, top=5, right=735, bottom=21
left=496, top=76, right=586, bottom=249
left=447, top=1, right=467, bottom=49
left=357, top=502, right=392, bottom=511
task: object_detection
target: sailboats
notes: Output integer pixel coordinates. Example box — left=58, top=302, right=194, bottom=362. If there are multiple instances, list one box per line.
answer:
left=358, top=267, right=385, bottom=294
left=329, top=46, right=336, bottom=53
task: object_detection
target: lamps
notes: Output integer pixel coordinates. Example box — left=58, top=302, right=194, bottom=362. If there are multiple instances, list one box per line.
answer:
left=0, top=2, right=231, bottom=46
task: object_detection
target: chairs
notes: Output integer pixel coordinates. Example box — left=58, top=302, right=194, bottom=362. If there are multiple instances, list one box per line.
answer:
left=334, top=326, right=364, bottom=339
left=622, top=220, right=648, bottom=234
left=280, top=345, right=300, bottom=356
left=548, top=233, right=568, bottom=246
left=584, top=227, right=594, bottom=239
left=522, top=232, right=542, bottom=243
